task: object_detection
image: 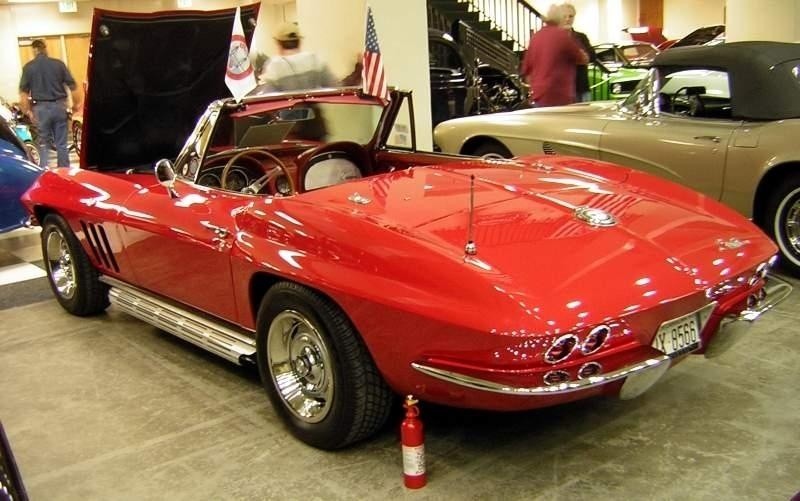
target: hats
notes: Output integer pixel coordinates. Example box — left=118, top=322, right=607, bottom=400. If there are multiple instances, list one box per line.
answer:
left=272, top=21, right=305, bottom=41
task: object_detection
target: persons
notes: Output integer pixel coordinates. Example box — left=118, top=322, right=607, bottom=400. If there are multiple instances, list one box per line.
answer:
left=20, top=40, right=82, bottom=169
left=259, top=24, right=364, bottom=95
left=518, top=4, right=588, bottom=108
left=558, top=3, right=619, bottom=104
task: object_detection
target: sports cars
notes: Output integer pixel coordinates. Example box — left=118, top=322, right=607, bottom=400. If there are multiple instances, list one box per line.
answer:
left=20, top=1, right=796, bottom=454
left=432, top=40, right=800, bottom=274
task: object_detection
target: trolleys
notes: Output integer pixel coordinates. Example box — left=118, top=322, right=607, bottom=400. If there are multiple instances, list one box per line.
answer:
left=1, top=97, right=82, bottom=167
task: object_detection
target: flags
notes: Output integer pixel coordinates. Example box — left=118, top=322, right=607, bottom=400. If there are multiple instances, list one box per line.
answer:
left=224, top=7, right=258, bottom=104
left=361, top=8, right=392, bottom=107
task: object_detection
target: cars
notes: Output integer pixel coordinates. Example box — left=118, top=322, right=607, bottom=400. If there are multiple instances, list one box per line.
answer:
left=584, top=68, right=649, bottom=101
left=0, top=115, right=43, bottom=233
left=584, top=25, right=731, bottom=97
